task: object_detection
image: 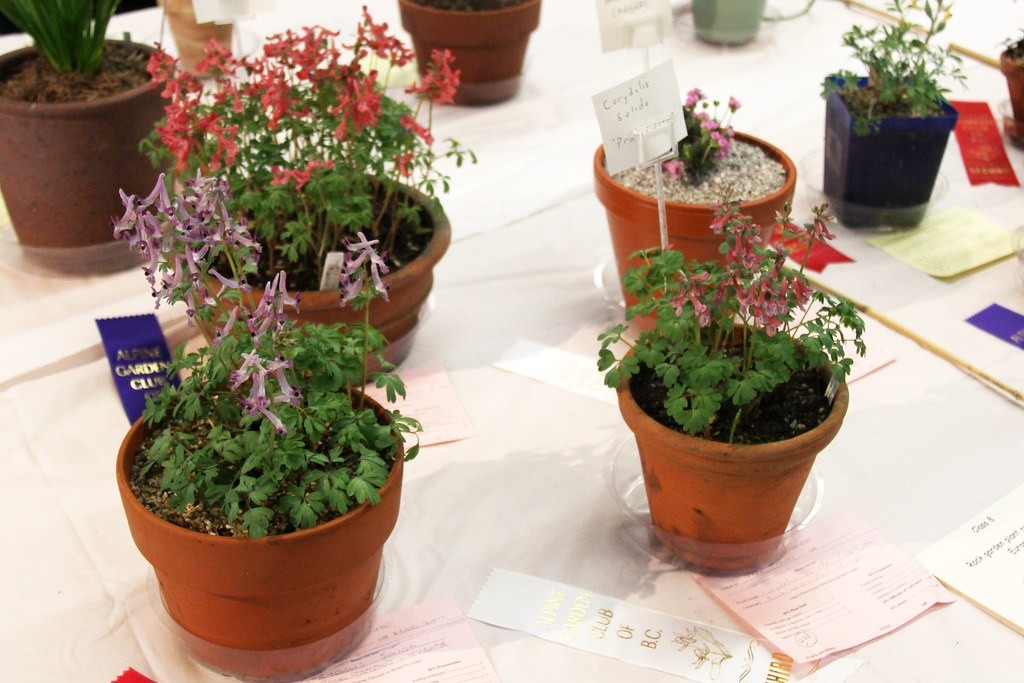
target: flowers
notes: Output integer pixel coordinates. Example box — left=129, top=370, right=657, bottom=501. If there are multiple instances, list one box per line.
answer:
left=135, top=4, right=481, bottom=278
left=665, top=88, right=744, bottom=186
left=820, top=0, right=967, bottom=135
left=109, top=169, right=424, bottom=538
left=594, top=187, right=869, bottom=442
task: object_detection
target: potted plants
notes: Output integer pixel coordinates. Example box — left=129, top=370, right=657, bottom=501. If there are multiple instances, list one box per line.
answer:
left=996, top=26, right=1024, bottom=151
left=0, top=0, right=178, bottom=254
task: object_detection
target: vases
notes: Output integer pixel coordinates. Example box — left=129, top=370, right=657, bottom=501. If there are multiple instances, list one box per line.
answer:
left=397, top=0, right=543, bottom=107
left=820, top=75, right=961, bottom=233
left=115, top=385, right=408, bottom=683
left=691, top=0, right=770, bottom=50
left=590, top=131, right=797, bottom=334
left=192, top=176, right=454, bottom=386
left=614, top=324, right=852, bottom=576
